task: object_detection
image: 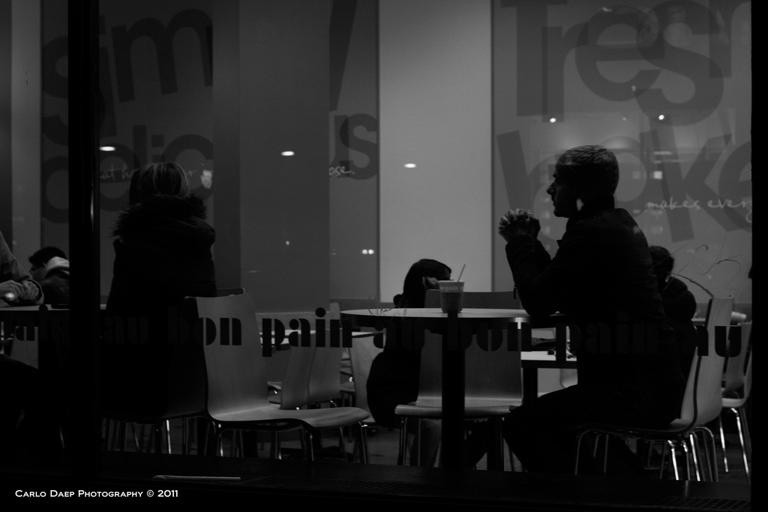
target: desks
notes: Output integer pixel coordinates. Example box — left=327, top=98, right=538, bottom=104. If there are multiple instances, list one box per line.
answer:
left=0, top=304, right=107, bottom=368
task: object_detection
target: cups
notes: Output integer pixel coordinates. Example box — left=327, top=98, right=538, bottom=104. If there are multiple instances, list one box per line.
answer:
left=437, top=280, right=465, bottom=313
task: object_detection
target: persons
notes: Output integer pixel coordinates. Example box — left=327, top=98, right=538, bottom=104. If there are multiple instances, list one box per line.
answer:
left=0, top=229, right=63, bottom=453
left=365, top=258, right=488, bottom=470
left=26, top=244, right=72, bottom=305
left=95, top=159, right=261, bottom=457
left=498, top=146, right=679, bottom=477
left=643, top=244, right=698, bottom=363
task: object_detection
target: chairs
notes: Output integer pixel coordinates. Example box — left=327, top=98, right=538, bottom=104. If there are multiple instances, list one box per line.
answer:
left=60, top=287, right=750, bottom=505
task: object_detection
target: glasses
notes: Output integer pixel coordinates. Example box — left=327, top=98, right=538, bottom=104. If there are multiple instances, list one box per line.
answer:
left=29, top=266, right=44, bottom=275
